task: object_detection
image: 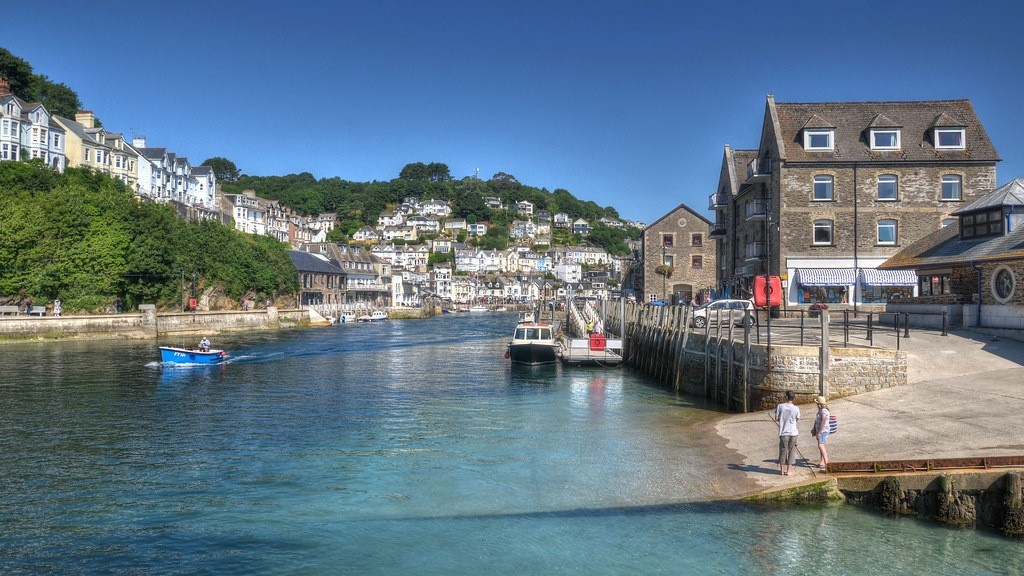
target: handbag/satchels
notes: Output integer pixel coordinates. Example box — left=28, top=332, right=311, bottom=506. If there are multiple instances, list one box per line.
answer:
left=828, top=415, right=837, bottom=433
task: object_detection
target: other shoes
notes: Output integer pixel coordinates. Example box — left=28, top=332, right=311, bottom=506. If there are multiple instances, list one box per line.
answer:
left=816, top=464, right=824, bottom=468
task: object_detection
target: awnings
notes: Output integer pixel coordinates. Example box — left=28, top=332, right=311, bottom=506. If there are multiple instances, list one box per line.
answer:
left=797, top=268, right=857, bottom=287
left=860, top=269, right=918, bottom=287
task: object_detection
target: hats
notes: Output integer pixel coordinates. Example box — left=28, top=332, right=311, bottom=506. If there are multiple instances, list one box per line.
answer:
left=814, top=396, right=827, bottom=405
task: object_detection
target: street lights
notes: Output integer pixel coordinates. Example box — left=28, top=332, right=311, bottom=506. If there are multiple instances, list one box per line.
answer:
left=180, top=273, right=196, bottom=312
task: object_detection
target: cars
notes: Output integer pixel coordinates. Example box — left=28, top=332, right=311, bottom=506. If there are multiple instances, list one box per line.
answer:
left=413, top=302, right=421, bottom=308
left=678, top=299, right=757, bottom=328
left=644, top=300, right=666, bottom=308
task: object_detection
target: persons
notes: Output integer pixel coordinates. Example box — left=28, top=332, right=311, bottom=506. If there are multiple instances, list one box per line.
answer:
left=587, top=321, right=595, bottom=338
left=775, top=391, right=800, bottom=475
left=54, top=297, right=61, bottom=317
left=594, top=322, right=603, bottom=335
left=814, top=396, right=830, bottom=468
left=200, top=337, right=211, bottom=353
left=26, top=299, right=33, bottom=316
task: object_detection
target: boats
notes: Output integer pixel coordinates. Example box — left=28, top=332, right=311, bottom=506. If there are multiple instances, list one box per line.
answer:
left=506, top=312, right=560, bottom=365
left=460, top=307, right=470, bottom=312
left=325, top=316, right=336, bottom=324
left=496, top=307, right=507, bottom=312
left=470, top=305, right=489, bottom=312
left=340, top=311, right=355, bottom=322
left=357, top=315, right=371, bottom=322
left=367, top=311, right=388, bottom=321
left=158, top=345, right=226, bottom=365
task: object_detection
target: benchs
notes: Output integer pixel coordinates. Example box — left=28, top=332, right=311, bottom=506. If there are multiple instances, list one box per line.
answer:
left=139, top=304, right=155, bottom=313
left=0, top=306, right=19, bottom=316
left=24, top=306, right=45, bottom=316
